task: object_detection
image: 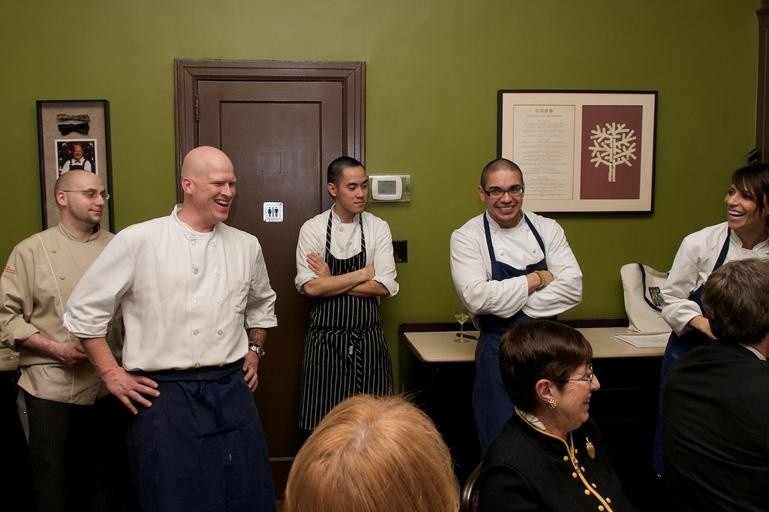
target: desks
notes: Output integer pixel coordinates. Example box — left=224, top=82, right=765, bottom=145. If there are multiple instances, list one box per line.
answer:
left=1, top=340, right=121, bottom=380
left=398, top=316, right=670, bottom=452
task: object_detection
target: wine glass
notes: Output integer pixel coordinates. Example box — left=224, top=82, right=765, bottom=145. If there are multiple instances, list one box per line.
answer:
left=452, top=299, right=470, bottom=343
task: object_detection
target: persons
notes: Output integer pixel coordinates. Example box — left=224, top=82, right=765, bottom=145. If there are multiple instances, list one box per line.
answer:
left=662, top=257, right=769, bottom=512
left=448, top=158, right=584, bottom=459
left=282, top=393, right=462, bottom=512
left=477, top=316, right=638, bottom=512
left=653, top=161, right=769, bottom=476
left=0, top=168, right=125, bottom=511
left=293, top=155, right=402, bottom=431
left=61, top=142, right=91, bottom=175
left=63, top=145, right=278, bottom=511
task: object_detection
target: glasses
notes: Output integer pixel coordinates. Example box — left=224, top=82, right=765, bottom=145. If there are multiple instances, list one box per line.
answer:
left=480, top=185, right=525, bottom=198
left=63, top=188, right=111, bottom=202
left=546, top=360, right=593, bottom=384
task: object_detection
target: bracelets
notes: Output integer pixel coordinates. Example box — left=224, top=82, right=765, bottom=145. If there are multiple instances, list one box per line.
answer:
left=533, top=269, right=546, bottom=294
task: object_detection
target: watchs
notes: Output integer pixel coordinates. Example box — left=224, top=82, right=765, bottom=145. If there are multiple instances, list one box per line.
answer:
left=244, top=339, right=269, bottom=360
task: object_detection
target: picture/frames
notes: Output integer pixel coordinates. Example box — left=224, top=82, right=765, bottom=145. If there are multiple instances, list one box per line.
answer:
left=35, top=98, right=116, bottom=234
left=494, top=87, right=658, bottom=221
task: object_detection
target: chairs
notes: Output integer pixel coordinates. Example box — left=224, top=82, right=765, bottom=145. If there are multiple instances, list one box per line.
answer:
left=460, top=460, right=484, bottom=510
